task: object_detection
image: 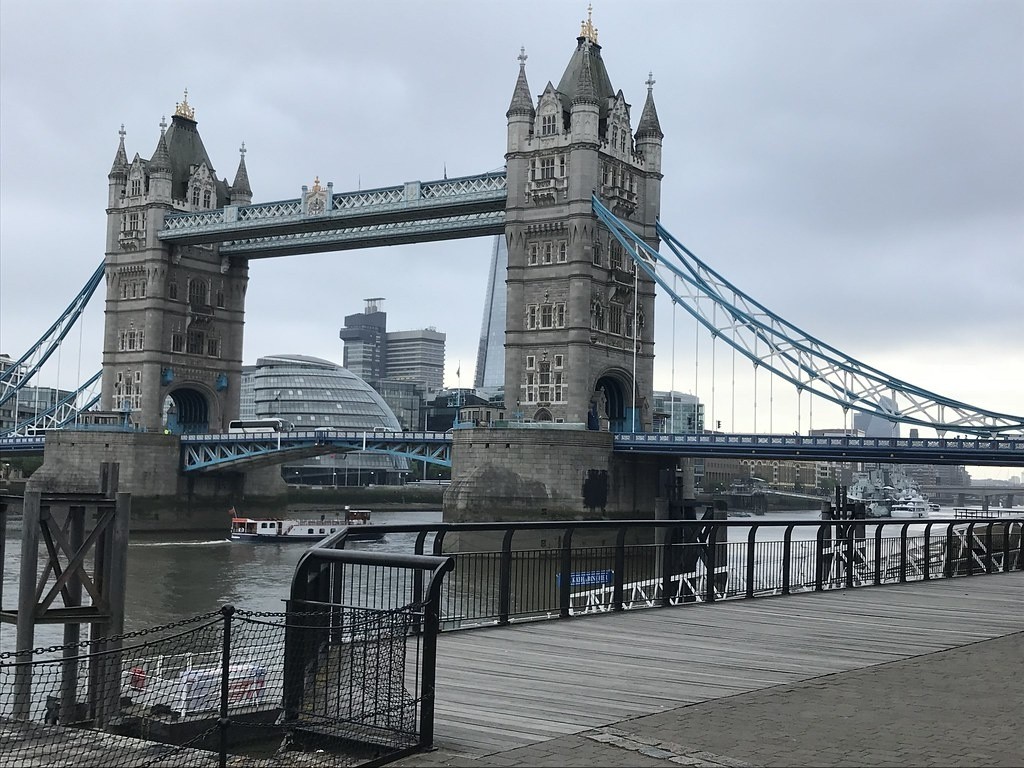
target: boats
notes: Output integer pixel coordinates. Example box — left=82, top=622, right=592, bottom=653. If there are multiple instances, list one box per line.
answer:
left=122, top=650, right=267, bottom=717
left=890, top=502, right=928, bottom=518
left=928, top=504, right=941, bottom=511
left=231, top=510, right=387, bottom=541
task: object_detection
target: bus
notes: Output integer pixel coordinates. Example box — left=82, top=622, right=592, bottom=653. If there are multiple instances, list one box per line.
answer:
left=228, top=418, right=294, bottom=433
